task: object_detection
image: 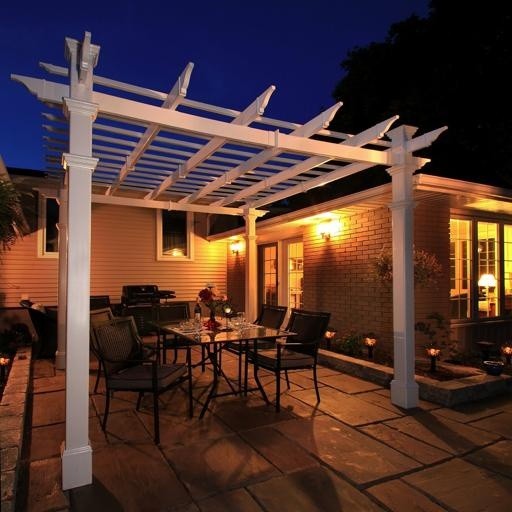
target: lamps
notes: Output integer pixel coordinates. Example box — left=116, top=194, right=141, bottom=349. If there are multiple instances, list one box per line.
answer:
left=364, top=332, right=376, bottom=359
left=316, top=217, right=348, bottom=245
left=500, top=341, right=512, bottom=364
left=478, top=273, right=497, bottom=318
left=426, top=343, right=440, bottom=369
left=227, top=239, right=245, bottom=260
left=324, top=326, right=337, bottom=348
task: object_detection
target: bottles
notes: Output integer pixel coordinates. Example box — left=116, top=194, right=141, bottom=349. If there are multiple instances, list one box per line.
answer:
left=194, top=297, right=202, bottom=321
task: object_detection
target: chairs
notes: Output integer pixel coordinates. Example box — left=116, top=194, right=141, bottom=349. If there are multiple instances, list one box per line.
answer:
left=90, top=307, right=115, bottom=393
left=20, top=300, right=57, bottom=376
left=157, top=303, right=205, bottom=372
left=93, top=315, right=193, bottom=444
left=122, top=304, right=157, bottom=358
left=244, top=308, right=331, bottom=413
left=219, top=304, right=288, bottom=397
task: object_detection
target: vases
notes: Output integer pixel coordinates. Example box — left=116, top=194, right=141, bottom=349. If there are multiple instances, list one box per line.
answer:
left=202, top=311, right=221, bottom=328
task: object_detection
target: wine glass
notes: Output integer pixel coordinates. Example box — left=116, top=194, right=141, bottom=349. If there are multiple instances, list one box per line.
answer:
left=231, top=317, right=241, bottom=336
left=237, top=312, right=245, bottom=326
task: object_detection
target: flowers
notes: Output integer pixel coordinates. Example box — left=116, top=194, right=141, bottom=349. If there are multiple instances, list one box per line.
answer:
left=198, top=288, right=228, bottom=311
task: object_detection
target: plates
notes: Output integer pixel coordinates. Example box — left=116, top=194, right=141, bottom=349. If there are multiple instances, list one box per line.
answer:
left=178, top=323, right=204, bottom=333
